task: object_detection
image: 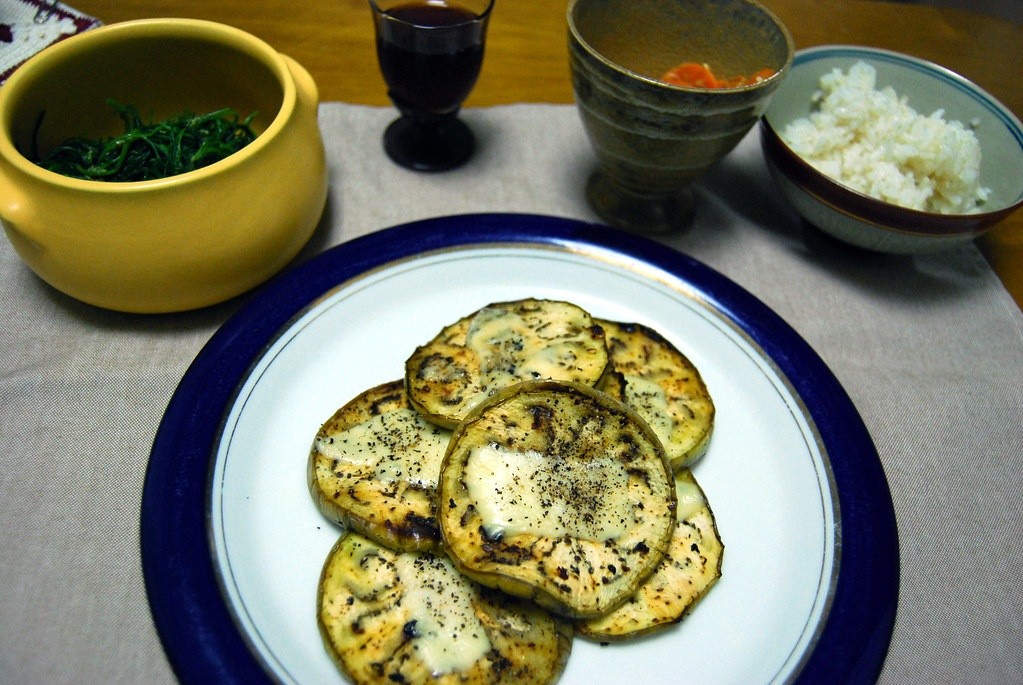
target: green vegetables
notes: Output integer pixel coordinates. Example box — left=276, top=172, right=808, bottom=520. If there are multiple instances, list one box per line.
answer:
left=25, top=97, right=261, bottom=182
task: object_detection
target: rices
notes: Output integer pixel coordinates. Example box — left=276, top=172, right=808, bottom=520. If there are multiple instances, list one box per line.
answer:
left=776, top=60, right=990, bottom=215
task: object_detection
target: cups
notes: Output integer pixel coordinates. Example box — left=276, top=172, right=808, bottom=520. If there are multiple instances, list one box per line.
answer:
left=368, top=0, right=496, bottom=173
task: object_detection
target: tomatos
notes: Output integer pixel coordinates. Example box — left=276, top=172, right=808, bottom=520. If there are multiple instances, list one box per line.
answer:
left=657, top=62, right=776, bottom=89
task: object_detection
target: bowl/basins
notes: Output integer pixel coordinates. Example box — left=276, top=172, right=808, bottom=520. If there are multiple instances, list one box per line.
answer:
left=566, top=0, right=795, bottom=239
left=1, top=16, right=331, bottom=314
left=758, top=44, right=1023, bottom=255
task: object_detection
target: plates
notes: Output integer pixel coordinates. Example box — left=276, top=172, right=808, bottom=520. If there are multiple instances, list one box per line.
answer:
left=205, top=237, right=844, bottom=685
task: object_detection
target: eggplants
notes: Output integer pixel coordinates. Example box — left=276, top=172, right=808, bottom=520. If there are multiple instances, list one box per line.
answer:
left=308, top=298, right=726, bottom=685
